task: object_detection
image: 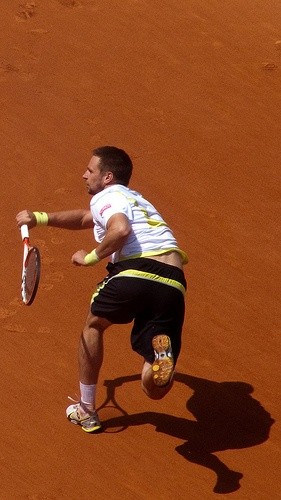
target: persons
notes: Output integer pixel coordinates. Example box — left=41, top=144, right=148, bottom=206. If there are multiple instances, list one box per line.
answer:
left=14, top=146, right=188, bottom=432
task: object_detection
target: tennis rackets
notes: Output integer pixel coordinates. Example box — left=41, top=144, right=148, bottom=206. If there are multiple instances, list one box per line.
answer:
left=20, top=224, right=41, bottom=306
